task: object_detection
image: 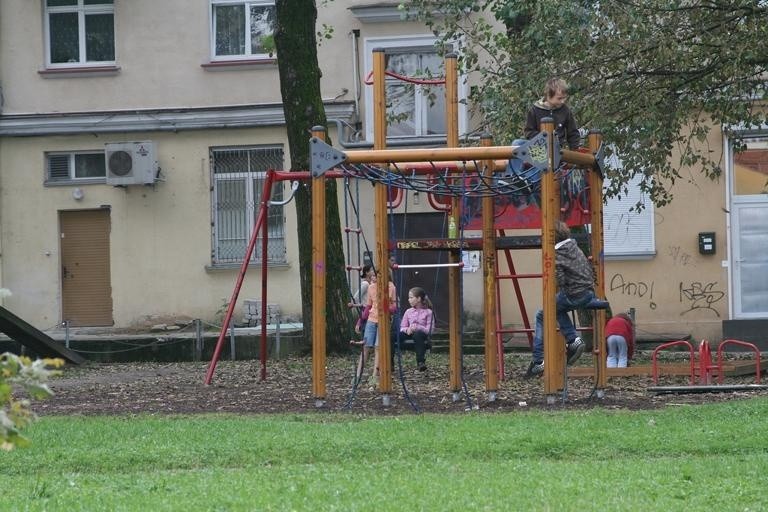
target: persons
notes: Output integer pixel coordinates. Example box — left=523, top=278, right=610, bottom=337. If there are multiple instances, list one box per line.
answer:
left=604, top=312, right=633, bottom=368
left=350, top=264, right=398, bottom=386
left=352, top=263, right=374, bottom=342
left=388, top=251, right=399, bottom=297
left=368, top=270, right=377, bottom=283
left=389, top=286, right=436, bottom=372
left=498, top=77, right=581, bottom=195
left=523, top=221, right=597, bottom=381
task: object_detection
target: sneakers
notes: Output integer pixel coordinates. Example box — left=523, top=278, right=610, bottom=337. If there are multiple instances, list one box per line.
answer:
left=369, top=376, right=377, bottom=384
left=497, top=176, right=534, bottom=195
left=351, top=377, right=361, bottom=384
left=418, top=362, right=427, bottom=372
left=525, top=360, right=544, bottom=378
left=567, top=336, right=586, bottom=365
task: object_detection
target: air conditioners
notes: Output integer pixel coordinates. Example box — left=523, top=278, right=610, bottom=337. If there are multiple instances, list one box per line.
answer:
left=104, top=140, right=158, bottom=185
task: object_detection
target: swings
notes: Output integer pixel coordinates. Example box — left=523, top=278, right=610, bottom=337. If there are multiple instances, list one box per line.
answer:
left=394, top=168, right=449, bottom=349
